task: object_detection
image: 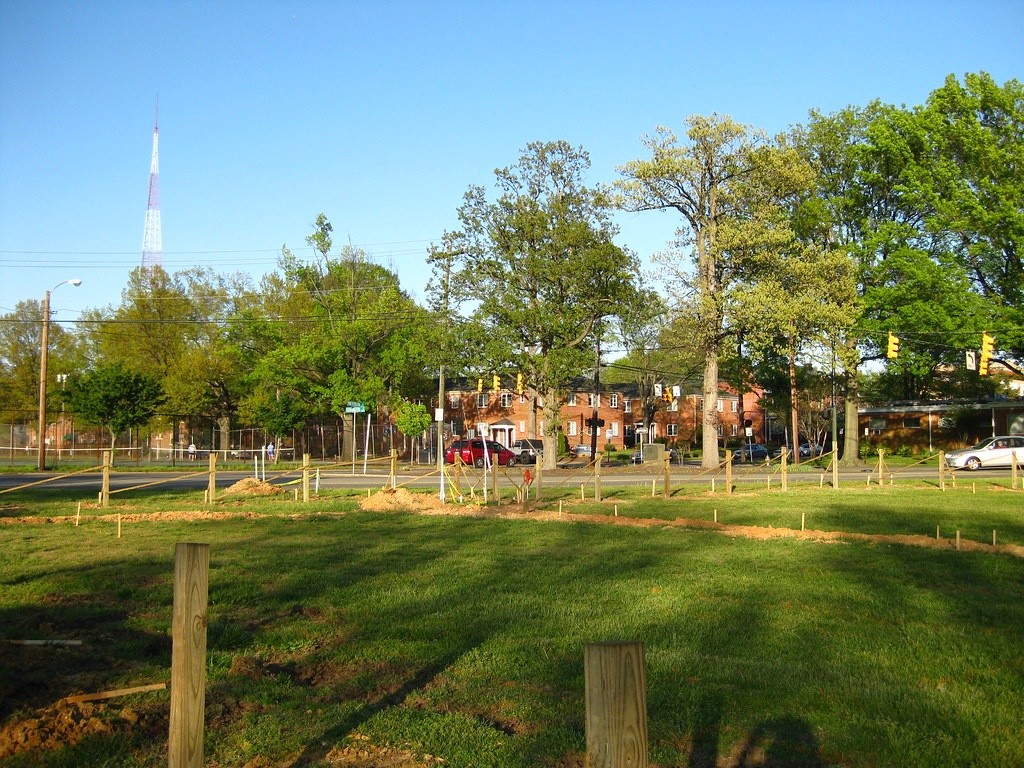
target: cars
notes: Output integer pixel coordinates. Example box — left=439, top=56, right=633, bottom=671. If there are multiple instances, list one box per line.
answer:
left=734, top=444, right=768, bottom=460
left=798, top=443, right=822, bottom=457
left=445, top=438, right=518, bottom=468
left=773, top=444, right=800, bottom=460
left=630, top=447, right=677, bottom=465
left=944, top=435, right=1024, bottom=471
left=230, top=443, right=251, bottom=459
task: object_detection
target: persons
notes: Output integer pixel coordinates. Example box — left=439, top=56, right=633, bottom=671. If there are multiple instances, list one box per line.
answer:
left=267, top=442, right=274, bottom=460
left=997, top=441, right=1007, bottom=448
left=188, top=443, right=196, bottom=461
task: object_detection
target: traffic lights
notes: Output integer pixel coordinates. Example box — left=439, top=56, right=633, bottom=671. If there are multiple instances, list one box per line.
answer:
left=982, top=333, right=994, bottom=359
left=979, top=357, right=989, bottom=376
left=662, top=387, right=672, bottom=404
left=478, top=378, right=484, bottom=393
left=517, top=373, right=522, bottom=390
left=494, top=375, right=501, bottom=390
left=887, top=334, right=899, bottom=359
left=519, top=387, right=524, bottom=395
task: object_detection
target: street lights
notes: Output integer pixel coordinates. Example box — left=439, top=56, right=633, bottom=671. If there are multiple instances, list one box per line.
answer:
left=37, top=280, right=83, bottom=470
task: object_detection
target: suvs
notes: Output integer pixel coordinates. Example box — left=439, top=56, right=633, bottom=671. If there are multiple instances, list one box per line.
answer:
left=508, top=438, right=544, bottom=464
